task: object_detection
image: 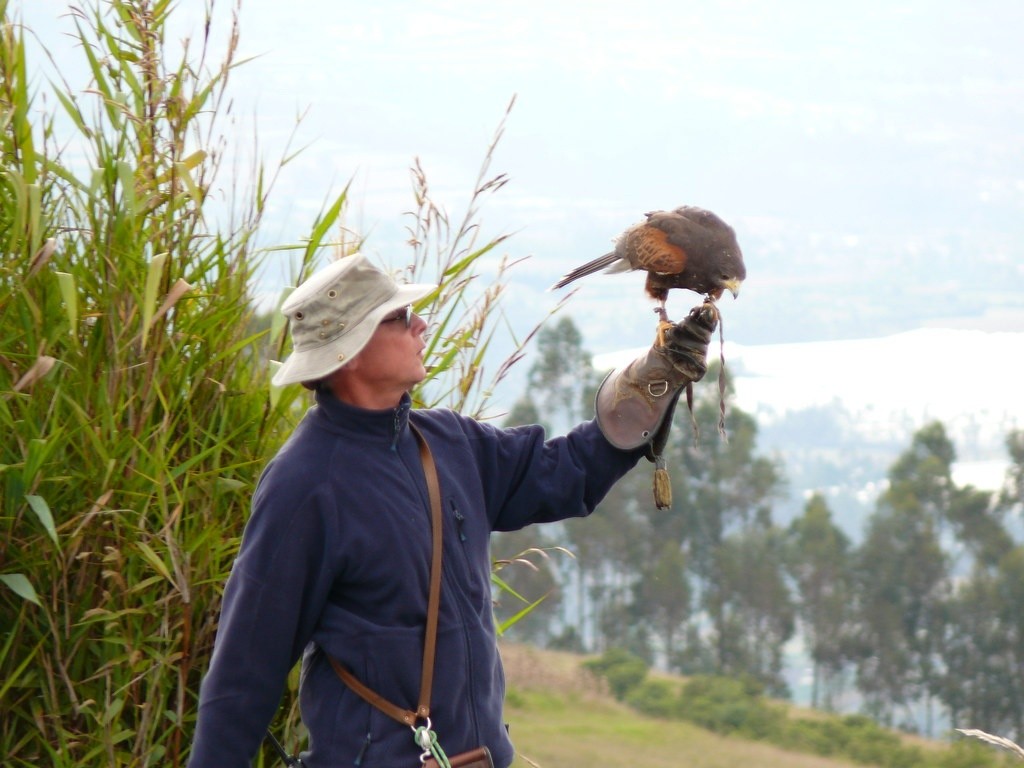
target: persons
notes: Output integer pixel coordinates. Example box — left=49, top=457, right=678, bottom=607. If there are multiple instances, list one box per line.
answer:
left=187, top=253, right=719, bottom=768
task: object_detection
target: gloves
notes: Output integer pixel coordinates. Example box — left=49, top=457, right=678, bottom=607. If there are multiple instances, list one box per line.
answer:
left=594, top=306, right=718, bottom=452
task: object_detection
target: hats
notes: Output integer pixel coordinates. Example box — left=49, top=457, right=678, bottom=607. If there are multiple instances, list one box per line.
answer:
left=271, top=254, right=441, bottom=387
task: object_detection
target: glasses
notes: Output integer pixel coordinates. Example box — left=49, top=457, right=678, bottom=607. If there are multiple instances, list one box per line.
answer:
left=380, top=305, right=413, bottom=329
